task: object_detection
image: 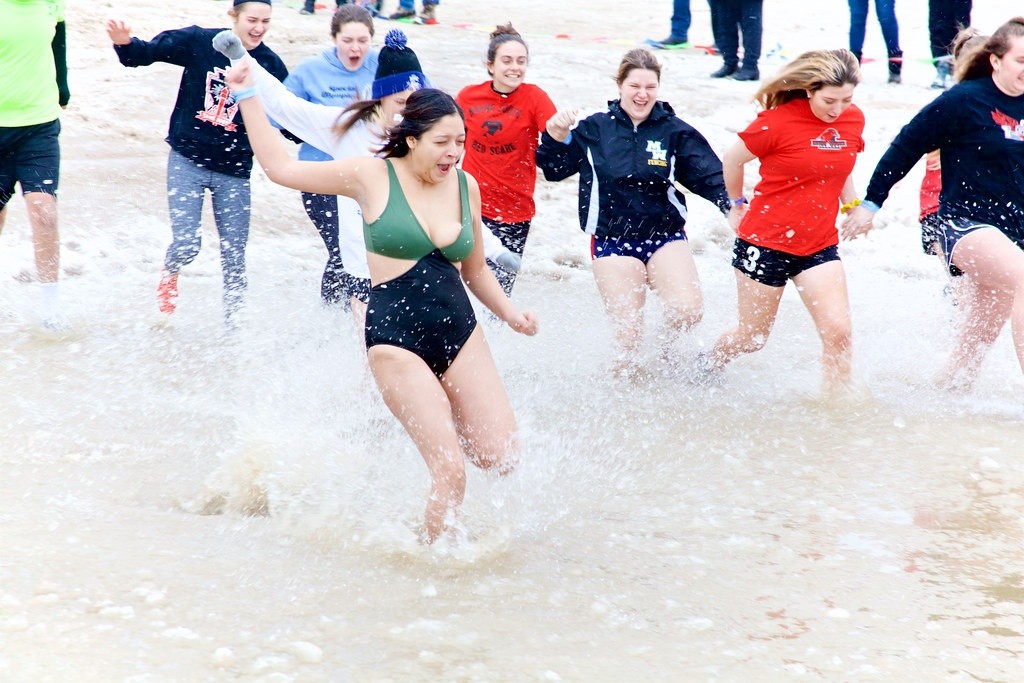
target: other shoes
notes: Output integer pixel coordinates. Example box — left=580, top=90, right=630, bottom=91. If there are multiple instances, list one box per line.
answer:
left=710, top=63, right=738, bottom=77
left=931, top=60, right=952, bottom=89
left=656, top=35, right=689, bottom=49
left=389, top=8, right=416, bottom=18
left=300, top=8, right=315, bottom=16
left=413, top=4, right=435, bottom=25
left=730, top=62, right=760, bottom=81
left=156, top=274, right=178, bottom=314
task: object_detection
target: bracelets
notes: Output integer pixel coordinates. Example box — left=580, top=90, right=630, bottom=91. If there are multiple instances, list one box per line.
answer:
left=564, top=132, right=572, bottom=145
left=232, top=87, right=257, bottom=101
left=730, top=197, right=745, bottom=206
left=861, top=200, right=880, bottom=212
left=841, top=199, right=862, bottom=214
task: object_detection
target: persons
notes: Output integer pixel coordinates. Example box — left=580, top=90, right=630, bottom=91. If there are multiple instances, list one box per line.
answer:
left=106, top=0, right=288, bottom=314
left=264, top=4, right=380, bottom=306
left=842, top=17, right=1024, bottom=378
left=709, top=48, right=864, bottom=382
left=927, top=0, right=972, bottom=88
left=226, top=62, right=541, bottom=544
left=396, top=0, right=440, bottom=23
left=454, top=23, right=558, bottom=297
left=212, top=28, right=430, bottom=350
left=919, top=25, right=990, bottom=279
left=659, top=0, right=763, bottom=81
left=847, top=0, right=903, bottom=83
left=535, top=48, right=748, bottom=354
left=0, top=0, right=71, bottom=285
left=301, top=0, right=348, bottom=17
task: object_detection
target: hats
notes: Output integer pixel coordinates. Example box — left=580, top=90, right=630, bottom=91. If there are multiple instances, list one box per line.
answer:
left=373, top=29, right=427, bottom=102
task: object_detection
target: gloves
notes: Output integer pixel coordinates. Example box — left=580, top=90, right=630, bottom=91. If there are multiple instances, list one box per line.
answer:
left=212, top=31, right=245, bottom=60
left=498, top=253, right=527, bottom=272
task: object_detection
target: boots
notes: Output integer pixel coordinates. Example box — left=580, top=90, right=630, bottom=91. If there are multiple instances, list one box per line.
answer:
left=887, top=50, right=904, bottom=84
